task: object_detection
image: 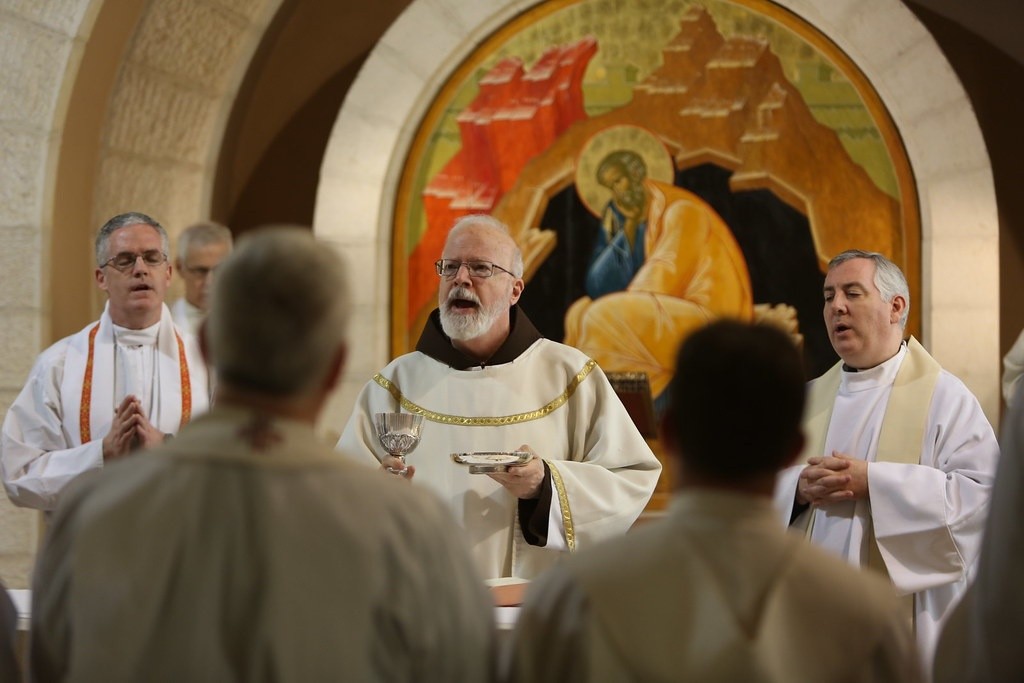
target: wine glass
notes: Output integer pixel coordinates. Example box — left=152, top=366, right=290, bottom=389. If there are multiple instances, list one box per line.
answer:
left=374, top=412, right=425, bottom=477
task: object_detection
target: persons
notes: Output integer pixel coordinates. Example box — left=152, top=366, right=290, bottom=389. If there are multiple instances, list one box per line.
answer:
left=169, top=222, right=234, bottom=410
left=773, top=250, right=1023, bottom=683
left=0, top=212, right=211, bottom=548
left=26, top=229, right=497, bottom=683
left=333, top=213, right=663, bottom=578
left=494, top=317, right=926, bottom=683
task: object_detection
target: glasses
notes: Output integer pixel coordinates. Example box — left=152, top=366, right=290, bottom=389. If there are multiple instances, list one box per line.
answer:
left=434, top=258, right=516, bottom=277
left=183, top=262, right=219, bottom=278
left=99, top=253, right=167, bottom=269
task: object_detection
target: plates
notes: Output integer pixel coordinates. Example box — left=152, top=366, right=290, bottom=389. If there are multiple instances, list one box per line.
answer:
left=450, top=450, right=534, bottom=474
